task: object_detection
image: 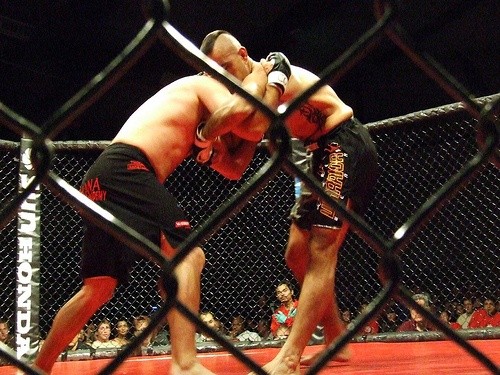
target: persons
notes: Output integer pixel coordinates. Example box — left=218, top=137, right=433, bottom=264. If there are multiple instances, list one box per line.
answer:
left=196, top=29, right=377, bottom=375
left=0, top=281, right=500, bottom=359
left=23, top=52, right=291, bottom=375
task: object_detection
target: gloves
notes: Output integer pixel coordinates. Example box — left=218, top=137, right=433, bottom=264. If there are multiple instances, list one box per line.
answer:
left=192, top=121, right=219, bottom=169
left=261, top=51, right=292, bottom=94
